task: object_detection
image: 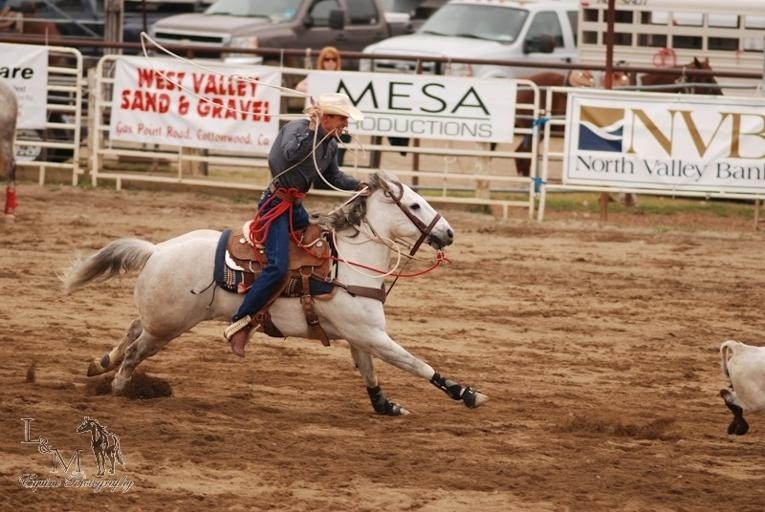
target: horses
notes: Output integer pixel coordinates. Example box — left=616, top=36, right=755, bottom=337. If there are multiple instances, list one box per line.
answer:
left=505, top=51, right=725, bottom=177
left=0, top=84, right=22, bottom=216
left=58, top=168, right=491, bottom=418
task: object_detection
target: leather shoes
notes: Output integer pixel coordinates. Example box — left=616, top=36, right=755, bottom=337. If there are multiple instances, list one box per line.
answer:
left=227, top=321, right=250, bottom=358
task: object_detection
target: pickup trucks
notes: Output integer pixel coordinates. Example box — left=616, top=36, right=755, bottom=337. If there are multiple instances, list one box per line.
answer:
left=147, top=0, right=427, bottom=95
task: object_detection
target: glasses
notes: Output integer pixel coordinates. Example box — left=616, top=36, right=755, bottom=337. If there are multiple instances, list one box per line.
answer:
left=323, top=57, right=336, bottom=61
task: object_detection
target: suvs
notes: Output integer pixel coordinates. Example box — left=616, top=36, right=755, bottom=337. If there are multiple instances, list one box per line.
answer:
left=356, top=1, right=579, bottom=91
left=1, top=0, right=214, bottom=163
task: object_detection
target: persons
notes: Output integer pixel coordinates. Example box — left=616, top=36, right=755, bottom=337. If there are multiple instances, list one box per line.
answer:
left=296, top=46, right=351, bottom=166
left=228, top=92, right=360, bottom=357
left=10, top=0, right=73, bottom=163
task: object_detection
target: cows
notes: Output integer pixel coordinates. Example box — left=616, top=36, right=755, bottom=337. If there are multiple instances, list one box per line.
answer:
left=716, top=339, right=765, bottom=434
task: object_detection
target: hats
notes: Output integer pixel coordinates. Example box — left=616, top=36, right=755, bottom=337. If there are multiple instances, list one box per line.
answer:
left=302, top=93, right=366, bottom=122
